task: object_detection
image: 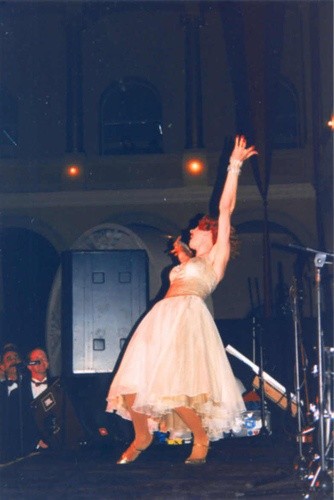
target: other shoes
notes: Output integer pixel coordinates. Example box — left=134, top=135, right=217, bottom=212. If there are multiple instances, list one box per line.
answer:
left=185, top=441, right=210, bottom=464
left=116, top=432, right=154, bottom=465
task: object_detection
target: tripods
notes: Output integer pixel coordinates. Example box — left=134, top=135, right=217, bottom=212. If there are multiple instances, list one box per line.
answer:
left=244, top=239, right=334, bottom=500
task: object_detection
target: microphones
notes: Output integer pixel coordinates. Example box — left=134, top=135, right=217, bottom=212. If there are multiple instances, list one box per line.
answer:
left=15, top=361, right=40, bottom=367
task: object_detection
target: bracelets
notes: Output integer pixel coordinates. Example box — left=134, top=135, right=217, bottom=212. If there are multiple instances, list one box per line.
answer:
left=227, top=165, right=240, bottom=172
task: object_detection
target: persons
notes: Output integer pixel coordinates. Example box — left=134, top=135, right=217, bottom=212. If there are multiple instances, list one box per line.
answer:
left=105, top=134, right=259, bottom=464
left=0, top=344, right=61, bottom=448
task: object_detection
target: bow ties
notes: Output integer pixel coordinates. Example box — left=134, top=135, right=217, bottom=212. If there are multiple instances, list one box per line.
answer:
left=6, top=379, right=19, bottom=386
left=31, top=379, right=48, bottom=386
